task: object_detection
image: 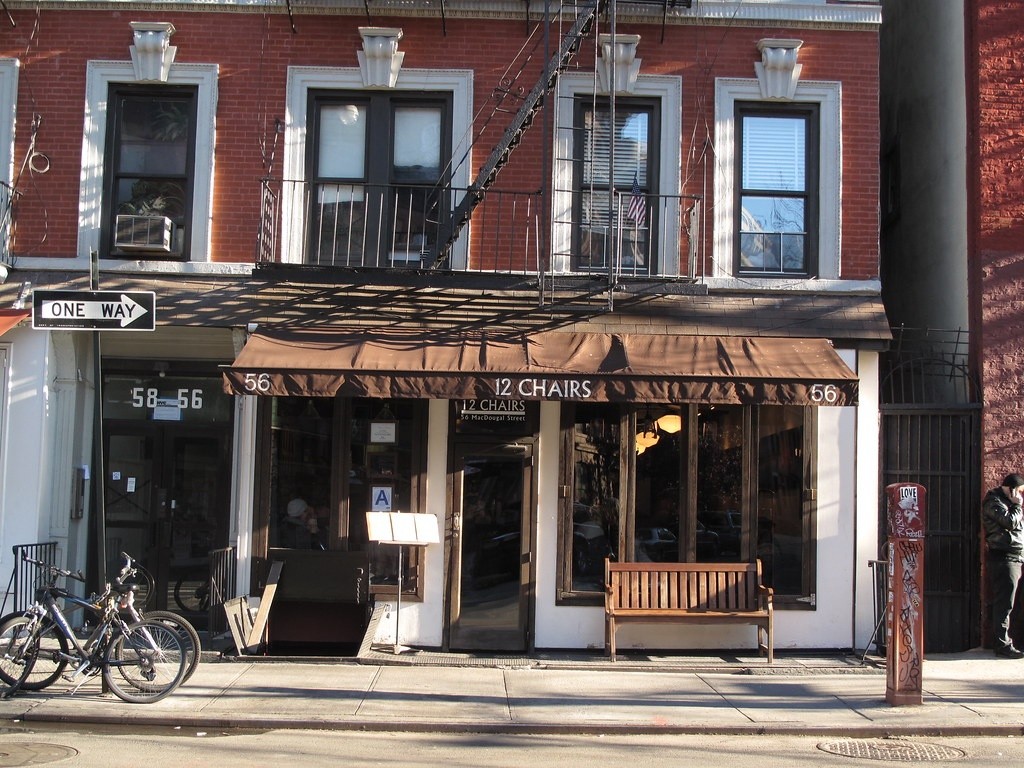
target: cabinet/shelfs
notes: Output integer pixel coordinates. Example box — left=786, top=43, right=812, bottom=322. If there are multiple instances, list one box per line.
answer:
left=364, top=447, right=412, bottom=585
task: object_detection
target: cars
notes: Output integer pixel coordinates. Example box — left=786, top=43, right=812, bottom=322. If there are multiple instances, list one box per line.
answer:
left=480, top=532, right=520, bottom=580
left=670, top=514, right=719, bottom=555
left=701, top=510, right=772, bottom=554
left=572, top=501, right=676, bottom=574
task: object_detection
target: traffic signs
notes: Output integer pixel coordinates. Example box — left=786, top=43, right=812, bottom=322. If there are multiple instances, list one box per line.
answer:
left=32, top=289, right=156, bottom=332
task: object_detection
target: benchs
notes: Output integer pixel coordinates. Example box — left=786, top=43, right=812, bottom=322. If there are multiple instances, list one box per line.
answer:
left=269, top=546, right=365, bottom=603
left=603, top=556, right=775, bottom=663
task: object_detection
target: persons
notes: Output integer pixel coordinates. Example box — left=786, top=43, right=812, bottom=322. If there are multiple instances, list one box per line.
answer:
left=982, top=473, right=1024, bottom=659
left=274, top=496, right=330, bottom=550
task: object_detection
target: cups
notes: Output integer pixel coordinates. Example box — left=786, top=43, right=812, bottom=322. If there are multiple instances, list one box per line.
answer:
left=308, top=519, right=317, bottom=534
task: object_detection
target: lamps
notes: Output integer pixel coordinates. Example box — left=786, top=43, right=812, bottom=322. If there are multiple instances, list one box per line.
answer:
left=634, top=413, right=683, bottom=455
left=337, top=105, right=359, bottom=127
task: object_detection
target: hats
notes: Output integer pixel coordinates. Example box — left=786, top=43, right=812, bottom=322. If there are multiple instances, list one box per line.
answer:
left=287, top=498, right=308, bottom=517
left=1003, top=473, right=1024, bottom=489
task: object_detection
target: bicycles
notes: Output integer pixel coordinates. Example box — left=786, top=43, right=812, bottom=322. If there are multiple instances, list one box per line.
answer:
left=110, top=561, right=155, bottom=612
left=0, top=550, right=201, bottom=704
left=174, top=540, right=238, bottom=614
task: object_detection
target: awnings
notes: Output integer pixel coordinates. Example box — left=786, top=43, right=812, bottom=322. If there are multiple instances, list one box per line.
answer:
left=221, top=326, right=859, bottom=408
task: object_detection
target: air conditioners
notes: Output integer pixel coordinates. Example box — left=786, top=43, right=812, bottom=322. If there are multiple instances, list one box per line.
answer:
left=114, top=214, right=173, bottom=252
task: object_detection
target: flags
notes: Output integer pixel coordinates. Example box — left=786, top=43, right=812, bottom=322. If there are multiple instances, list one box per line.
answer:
left=627, top=172, right=646, bottom=224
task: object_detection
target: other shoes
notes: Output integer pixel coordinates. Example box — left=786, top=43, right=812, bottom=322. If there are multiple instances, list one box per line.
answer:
left=996, top=649, right=1024, bottom=659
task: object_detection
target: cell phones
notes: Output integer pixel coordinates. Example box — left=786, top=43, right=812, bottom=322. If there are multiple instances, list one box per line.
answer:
left=1009, top=486, right=1016, bottom=498
